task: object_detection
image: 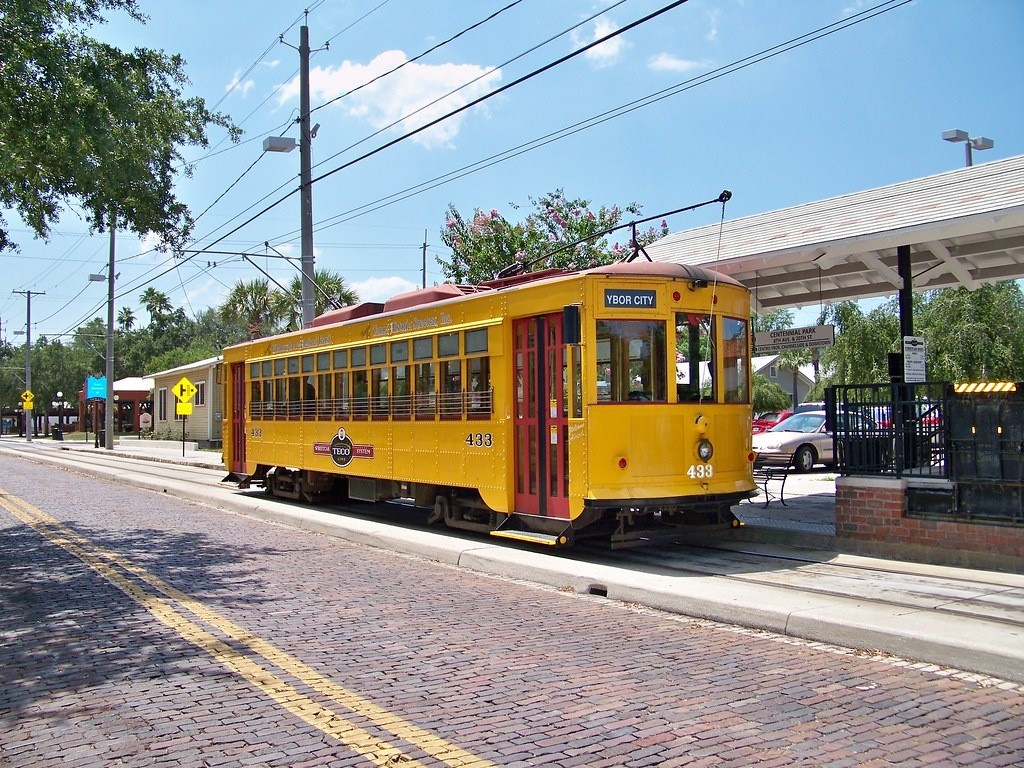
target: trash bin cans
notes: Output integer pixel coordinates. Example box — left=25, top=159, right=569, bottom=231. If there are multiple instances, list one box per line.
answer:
left=99, top=430, right=105, bottom=447
left=52, top=428, right=62, bottom=440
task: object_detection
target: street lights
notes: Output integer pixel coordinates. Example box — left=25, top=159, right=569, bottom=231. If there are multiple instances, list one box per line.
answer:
left=14, top=331, right=32, bottom=441
left=18, top=402, right=22, bottom=437
left=942, top=129, right=993, bottom=167
left=57, top=392, right=63, bottom=440
left=263, top=136, right=315, bottom=329
left=88, top=274, right=114, bottom=450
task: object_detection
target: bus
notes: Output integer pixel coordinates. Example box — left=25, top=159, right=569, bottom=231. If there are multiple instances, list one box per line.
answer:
left=215, top=263, right=759, bottom=554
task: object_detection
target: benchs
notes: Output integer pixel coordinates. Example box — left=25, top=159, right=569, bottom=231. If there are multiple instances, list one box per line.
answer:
left=747, top=452, right=794, bottom=509
left=51, top=425, right=75, bottom=432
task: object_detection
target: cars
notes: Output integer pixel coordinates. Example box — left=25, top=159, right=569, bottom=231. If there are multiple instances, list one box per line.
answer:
left=794, top=402, right=844, bottom=423
left=752, top=412, right=794, bottom=435
left=752, top=410, right=895, bottom=473
